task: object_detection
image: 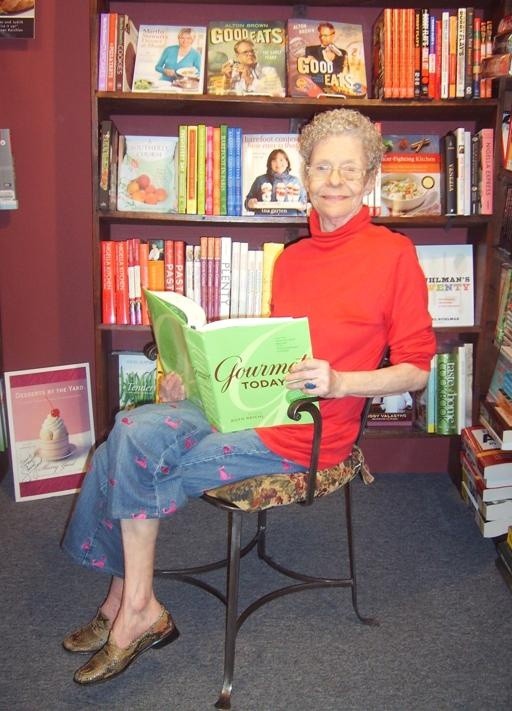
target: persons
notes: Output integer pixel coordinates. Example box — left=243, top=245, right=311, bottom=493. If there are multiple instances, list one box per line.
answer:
left=60, top=108, right=437, bottom=685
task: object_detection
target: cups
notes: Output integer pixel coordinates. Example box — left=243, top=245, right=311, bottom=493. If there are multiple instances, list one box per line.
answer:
left=261, top=181, right=301, bottom=203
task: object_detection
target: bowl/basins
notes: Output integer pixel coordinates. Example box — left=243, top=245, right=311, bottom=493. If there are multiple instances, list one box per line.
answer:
left=381, top=173, right=427, bottom=211
left=331, top=85, right=366, bottom=99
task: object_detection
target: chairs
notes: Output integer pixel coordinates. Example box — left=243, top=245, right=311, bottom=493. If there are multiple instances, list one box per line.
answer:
left=141, top=339, right=394, bottom=709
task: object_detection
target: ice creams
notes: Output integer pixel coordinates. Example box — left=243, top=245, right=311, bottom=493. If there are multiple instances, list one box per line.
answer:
left=41, top=407, right=67, bottom=440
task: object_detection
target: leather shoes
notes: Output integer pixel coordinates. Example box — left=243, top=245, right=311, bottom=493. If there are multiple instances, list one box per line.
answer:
left=61, top=605, right=110, bottom=652
left=72, top=604, right=181, bottom=686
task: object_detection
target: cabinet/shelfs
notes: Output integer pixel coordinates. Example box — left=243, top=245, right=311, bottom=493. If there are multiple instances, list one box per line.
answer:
left=89, top=0, right=495, bottom=472
left=469, top=74, right=512, bottom=594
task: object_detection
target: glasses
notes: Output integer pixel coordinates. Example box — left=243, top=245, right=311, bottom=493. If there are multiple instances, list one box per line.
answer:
left=301, top=164, right=371, bottom=183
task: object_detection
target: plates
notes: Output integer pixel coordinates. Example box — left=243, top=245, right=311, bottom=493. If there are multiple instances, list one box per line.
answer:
left=176, top=68, right=198, bottom=76
left=33, top=444, right=77, bottom=461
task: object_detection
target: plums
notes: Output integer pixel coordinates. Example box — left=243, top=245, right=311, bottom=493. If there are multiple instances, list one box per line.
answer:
left=128, top=175, right=166, bottom=204
